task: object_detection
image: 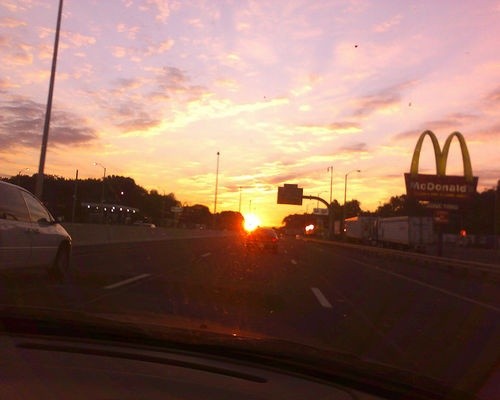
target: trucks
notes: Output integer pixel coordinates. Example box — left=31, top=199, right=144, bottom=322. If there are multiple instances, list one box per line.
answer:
left=342, top=217, right=375, bottom=246
left=375, top=214, right=434, bottom=256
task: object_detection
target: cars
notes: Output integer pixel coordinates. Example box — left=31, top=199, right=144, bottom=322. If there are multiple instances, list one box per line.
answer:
left=247, top=227, right=278, bottom=253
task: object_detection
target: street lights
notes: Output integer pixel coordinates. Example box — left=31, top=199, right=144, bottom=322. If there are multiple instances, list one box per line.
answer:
left=344, top=169, right=361, bottom=221
left=316, top=190, right=327, bottom=209
left=92, top=161, right=107, bottom=204
left=326, top=166, right=333, bottom=205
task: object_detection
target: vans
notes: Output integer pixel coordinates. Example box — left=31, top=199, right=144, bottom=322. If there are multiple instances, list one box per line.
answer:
left=1, top=181, right=72, bottom=281
